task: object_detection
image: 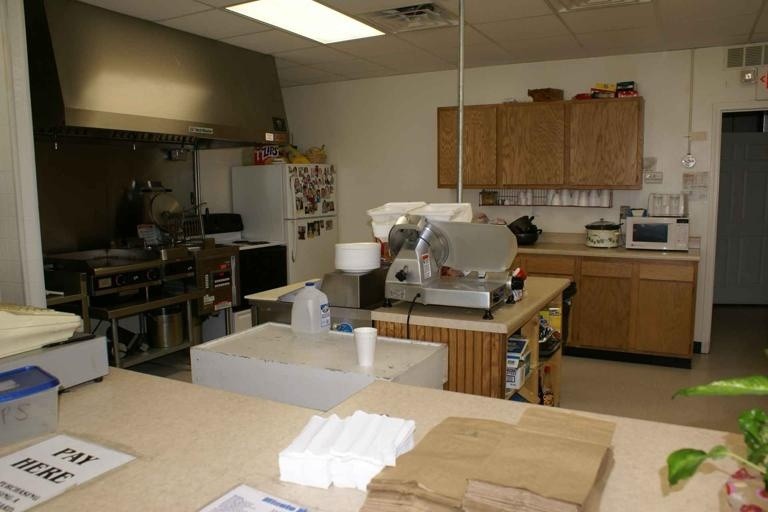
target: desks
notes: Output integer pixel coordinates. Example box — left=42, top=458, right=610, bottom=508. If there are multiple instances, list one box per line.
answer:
left=189, top=320, right=449, bottom=412
left=371, top=275, right=571, bottom=408
left=1, top=365, right=746, bottom=512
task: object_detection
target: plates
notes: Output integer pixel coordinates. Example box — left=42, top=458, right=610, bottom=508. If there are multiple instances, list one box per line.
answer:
left=333, top=242, right=381, bottom=274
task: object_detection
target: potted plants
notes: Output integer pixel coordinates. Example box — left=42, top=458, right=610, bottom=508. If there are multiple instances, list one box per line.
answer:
left=666, top=375, right=768, bottom=512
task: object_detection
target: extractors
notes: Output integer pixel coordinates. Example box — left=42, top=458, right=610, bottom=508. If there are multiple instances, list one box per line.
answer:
left=28, top=2, right=292, bottom=152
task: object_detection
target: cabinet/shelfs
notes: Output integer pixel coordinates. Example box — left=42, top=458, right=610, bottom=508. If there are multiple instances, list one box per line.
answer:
left=509, top=254, right=701, bottom=370
left=436, top=95, right=645, bottom=190
left=45, top=269, right=92, bottom=335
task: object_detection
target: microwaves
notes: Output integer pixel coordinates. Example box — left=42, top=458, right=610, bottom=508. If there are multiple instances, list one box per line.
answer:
left=626, top=216, right=691, bottom=252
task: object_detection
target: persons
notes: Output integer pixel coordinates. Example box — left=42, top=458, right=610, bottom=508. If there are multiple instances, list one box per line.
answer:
left=293, top=165, right=336, bottom=240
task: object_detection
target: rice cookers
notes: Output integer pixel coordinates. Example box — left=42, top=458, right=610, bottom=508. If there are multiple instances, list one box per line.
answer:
left=586, top=218, right=621, bottom=248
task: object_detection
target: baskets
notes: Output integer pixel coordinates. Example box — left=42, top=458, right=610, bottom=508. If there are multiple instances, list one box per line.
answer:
left=306, top=150, right=327, bottom=164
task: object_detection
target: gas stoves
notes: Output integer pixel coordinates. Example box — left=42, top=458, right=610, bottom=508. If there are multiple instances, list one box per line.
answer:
left=214, top=238, right=284, bottom=252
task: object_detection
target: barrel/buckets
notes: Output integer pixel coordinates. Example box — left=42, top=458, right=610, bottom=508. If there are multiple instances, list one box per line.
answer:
left=290, top=282, right=331, bottom=334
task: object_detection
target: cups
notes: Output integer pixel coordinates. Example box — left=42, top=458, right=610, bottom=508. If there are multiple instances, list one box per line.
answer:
left=481, top=188, right=612, bottom=207
left=632, top=210, right=646, bottom=216
left=354, top=326, right=377, bottom=367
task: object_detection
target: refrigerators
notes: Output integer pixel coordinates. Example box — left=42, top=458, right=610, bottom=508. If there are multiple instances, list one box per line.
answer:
left=230, top=163, right=339, bottom=284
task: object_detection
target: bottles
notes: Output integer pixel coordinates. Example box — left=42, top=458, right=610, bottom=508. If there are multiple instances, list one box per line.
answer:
left=127, top=175, right=155, bottom=192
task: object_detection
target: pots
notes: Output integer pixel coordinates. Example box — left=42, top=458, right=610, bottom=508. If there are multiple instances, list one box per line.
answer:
left=510, top=215, right=543, bottom=244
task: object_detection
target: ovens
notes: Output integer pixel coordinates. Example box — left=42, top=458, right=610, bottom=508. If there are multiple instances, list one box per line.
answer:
left=231, top=247, right=287, bottom=334
